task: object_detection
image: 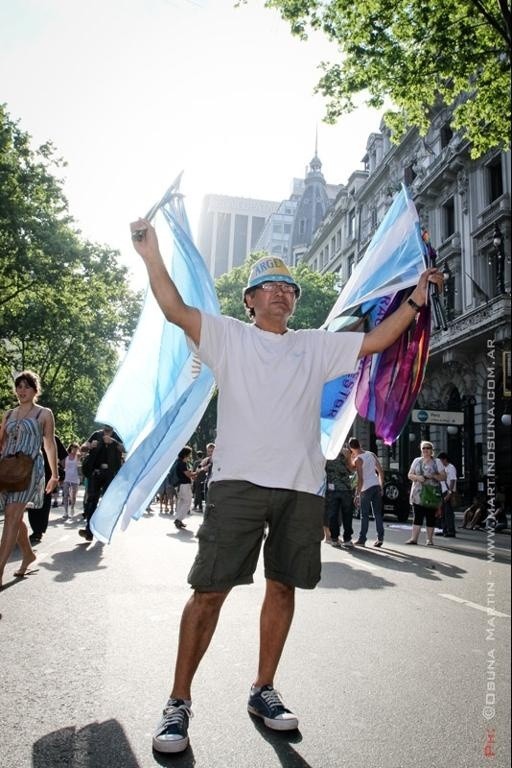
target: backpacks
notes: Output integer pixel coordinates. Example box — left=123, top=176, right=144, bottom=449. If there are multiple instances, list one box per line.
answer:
left=167, top=460, right=182, bottom=492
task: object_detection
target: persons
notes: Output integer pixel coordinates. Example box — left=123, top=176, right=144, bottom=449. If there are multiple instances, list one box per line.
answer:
left=148, top=439, right=214, bottom=530
left=120, top=217, right=445, bottom=753
left=324, top=439, right=507, bottom=546
left=0, top=369, right=60, bottom=590
left=28, top=425, right=126, bottom=547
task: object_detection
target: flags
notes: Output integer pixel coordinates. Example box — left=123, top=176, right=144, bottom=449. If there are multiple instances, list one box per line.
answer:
left=315, top=187, right=436, bottom=464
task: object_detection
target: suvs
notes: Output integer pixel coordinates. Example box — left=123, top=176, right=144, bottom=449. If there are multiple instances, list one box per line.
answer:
left=351, top=467, right=410, bottom=523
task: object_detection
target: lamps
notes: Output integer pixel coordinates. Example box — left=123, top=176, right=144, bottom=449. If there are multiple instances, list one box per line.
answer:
left=501, top=407, right=512, bottom=426
left=446, top=425, right=459, bottom=435
left=492, top=220, right=503, bottom=247
left=442, top=260, right=452, bottom=280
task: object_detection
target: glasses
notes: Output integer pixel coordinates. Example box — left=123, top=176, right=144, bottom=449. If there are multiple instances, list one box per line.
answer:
left=256, top=282, right=298, bottom=294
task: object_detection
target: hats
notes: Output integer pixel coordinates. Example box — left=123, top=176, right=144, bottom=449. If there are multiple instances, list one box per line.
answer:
left=242, top=255, right=301, bottom=300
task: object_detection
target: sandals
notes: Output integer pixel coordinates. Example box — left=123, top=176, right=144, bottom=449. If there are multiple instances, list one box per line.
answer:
left=14, top=548, right=41, bottom=577
left=405, top=537, right=433, bottom=545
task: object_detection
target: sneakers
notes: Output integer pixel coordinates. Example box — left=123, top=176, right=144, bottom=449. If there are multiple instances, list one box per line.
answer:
left=29, top=531, right=44, bottom=545
left=174, top=518, right=187, bottom=530
left=246, top=684, right=299, bottom=732
left=324, top=536, right=383, bottom=548
left=78, top=526, right=95, bottom=544
left=152, top=697, right=194, bottom=753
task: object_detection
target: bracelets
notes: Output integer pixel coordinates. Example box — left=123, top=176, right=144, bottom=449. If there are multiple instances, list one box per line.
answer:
left=408, top=297, right=423, bottom=314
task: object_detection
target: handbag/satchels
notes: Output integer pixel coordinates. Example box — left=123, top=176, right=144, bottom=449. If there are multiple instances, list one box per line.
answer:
left=0, top=451, right=35, bottom=493
left=419, top=484, right=443, bottom=509
